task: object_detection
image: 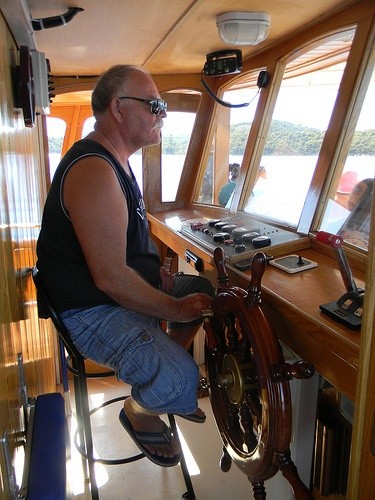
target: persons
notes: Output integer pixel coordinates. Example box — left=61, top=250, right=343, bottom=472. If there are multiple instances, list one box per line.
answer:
left=31, top=63, right=217, bottom=467
left=197, top=148, right=266, bottom=209
left=319, top=171, right=375, bottom=236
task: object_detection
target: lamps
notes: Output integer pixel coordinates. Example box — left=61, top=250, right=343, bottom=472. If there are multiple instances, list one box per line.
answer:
left=216, top=11, right=270, bottom=45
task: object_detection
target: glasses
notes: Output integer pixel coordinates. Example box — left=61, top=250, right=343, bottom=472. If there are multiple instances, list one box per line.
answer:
left=118, top=96, right=168, bottom=114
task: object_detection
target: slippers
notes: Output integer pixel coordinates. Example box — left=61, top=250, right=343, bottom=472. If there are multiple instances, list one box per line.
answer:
left=119, top=407, right=183, bottom=467
left=175, top=406, right=207, bottom=424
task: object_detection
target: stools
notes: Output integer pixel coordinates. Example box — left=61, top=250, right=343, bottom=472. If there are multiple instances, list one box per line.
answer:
left=33, top=265, right=196, bottom=500
